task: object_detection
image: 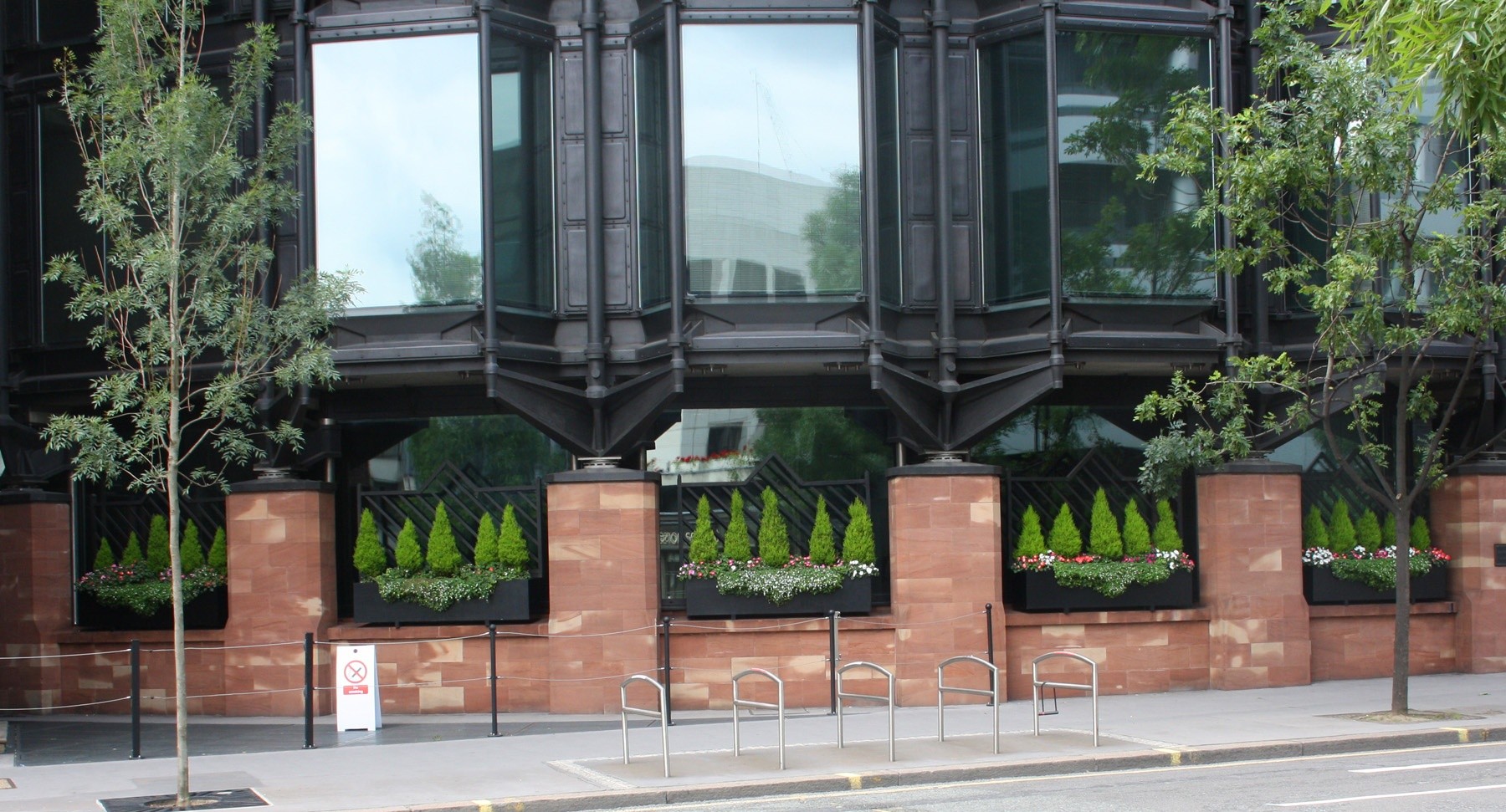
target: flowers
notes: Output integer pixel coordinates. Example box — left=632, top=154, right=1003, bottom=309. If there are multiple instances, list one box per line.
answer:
left=1301, top=544, right=1452, bottom=593
left=675, top=555, right=880, bottom=607
left=73, top=559, right=228, bottom=618
left=1009, top=549, right=1195, bottom=599
left=359, top=563, right=531, bottom=612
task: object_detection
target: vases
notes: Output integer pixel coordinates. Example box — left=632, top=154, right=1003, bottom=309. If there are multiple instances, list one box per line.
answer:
left=1304, top=566, right=1451, bottom=604
left=76, top=586, right=228, bottom=631
left=1011, top=567, right=1195, bottom=614
left=684, top=575, right=872, bottom=621
left=352, top=580, right=529, bottom=630
